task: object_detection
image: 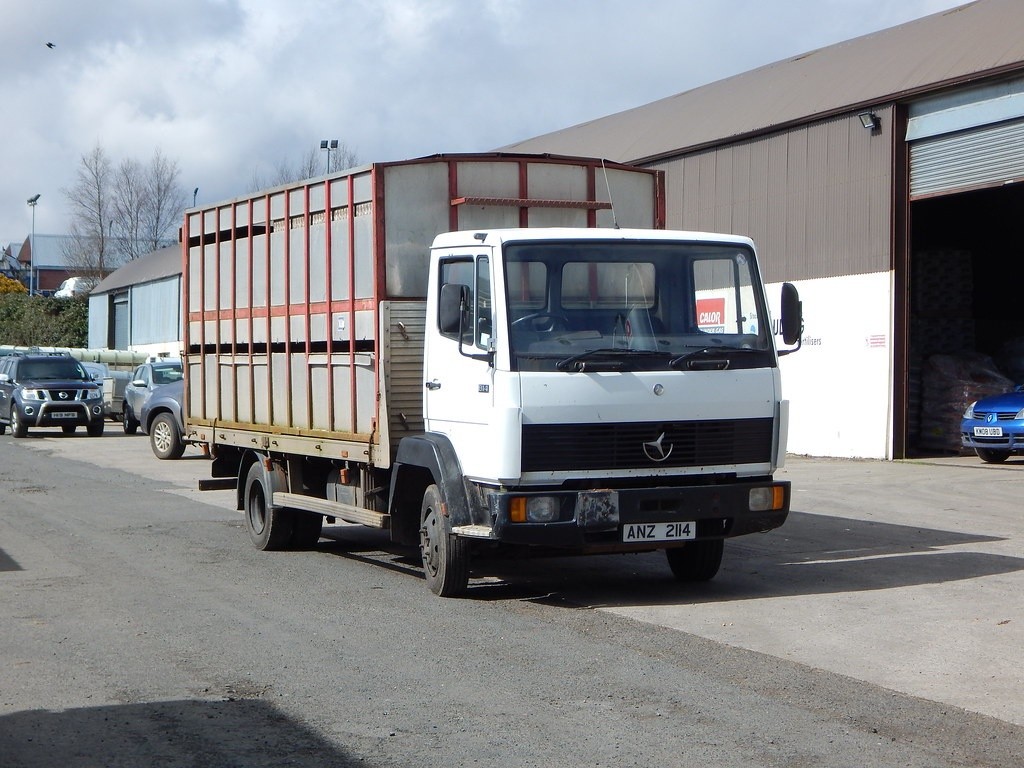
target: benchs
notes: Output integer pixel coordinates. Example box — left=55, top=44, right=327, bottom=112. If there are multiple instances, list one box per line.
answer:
left=548, top=316, right=666, bottom=336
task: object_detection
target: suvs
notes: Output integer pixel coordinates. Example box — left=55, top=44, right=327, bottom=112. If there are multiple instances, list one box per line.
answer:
left=0, top=346, right=105, bottom=438
left=121, top=356, right=200, bottom=460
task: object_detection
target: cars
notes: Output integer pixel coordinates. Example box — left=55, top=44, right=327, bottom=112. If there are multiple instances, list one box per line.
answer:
left=34, top=288, right=56, bottom=298
left=960, top=385, right=1024, bottom=463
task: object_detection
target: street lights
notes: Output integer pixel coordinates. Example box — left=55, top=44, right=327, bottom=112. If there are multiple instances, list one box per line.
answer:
left=27, top=194, right=42, bottom=297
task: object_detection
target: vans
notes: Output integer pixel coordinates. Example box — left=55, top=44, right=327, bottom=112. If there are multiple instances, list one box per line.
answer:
left=53, top=277, right=102, bottom=300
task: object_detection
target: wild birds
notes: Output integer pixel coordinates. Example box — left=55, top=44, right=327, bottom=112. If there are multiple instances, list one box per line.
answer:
left=46, top=42, right=56, bottom=49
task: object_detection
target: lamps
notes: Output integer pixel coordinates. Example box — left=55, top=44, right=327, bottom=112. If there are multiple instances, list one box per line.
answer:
left=858, top=112, right=881, bottom=129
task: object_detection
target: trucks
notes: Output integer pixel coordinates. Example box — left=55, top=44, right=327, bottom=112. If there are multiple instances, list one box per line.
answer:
left=182, top=152, right=804, bottom=598
